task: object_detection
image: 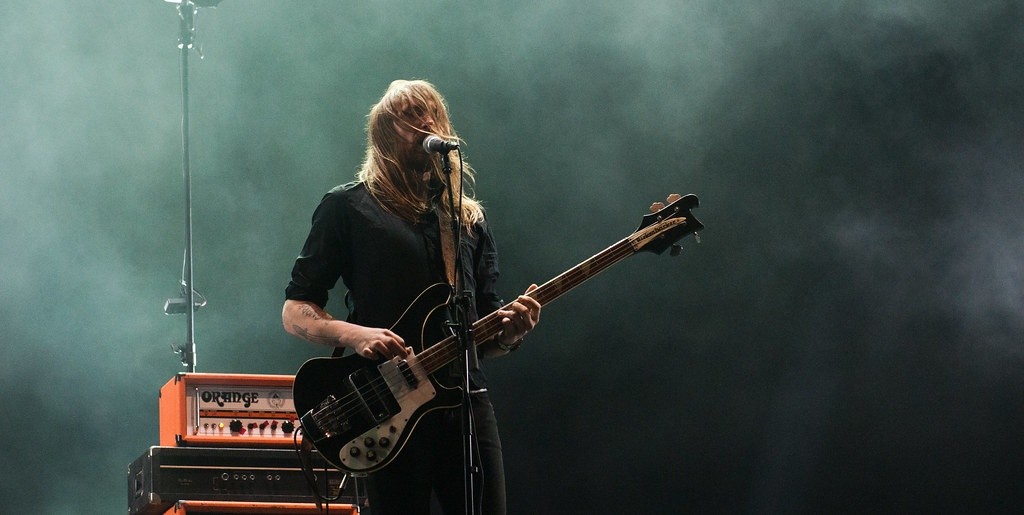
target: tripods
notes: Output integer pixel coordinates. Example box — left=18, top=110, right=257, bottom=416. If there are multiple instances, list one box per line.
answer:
left=439, top=141, right=478, bottom=514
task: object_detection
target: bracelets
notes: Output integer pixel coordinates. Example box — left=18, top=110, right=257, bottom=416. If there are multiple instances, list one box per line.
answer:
left=494, top=332, right=523, bottom=353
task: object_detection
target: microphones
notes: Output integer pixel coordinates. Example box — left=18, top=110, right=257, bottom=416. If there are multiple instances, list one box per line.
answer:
left=423, top=135, right=457, bottom=154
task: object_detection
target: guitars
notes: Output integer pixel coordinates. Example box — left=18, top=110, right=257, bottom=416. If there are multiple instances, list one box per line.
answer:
left=294, top=194, right=708, bottom=477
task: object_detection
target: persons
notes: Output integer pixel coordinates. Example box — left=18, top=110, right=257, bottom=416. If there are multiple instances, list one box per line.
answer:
left=282, top=78, right=541, bottom=515
